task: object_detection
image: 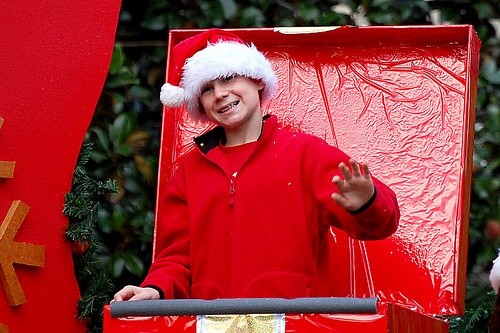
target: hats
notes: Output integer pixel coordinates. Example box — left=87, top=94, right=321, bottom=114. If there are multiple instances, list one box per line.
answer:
left=160, top=28, right=279, bottom=122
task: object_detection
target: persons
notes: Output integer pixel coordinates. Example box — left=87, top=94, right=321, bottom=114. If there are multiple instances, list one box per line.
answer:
left=109, top=29, right=401, bottom=301
